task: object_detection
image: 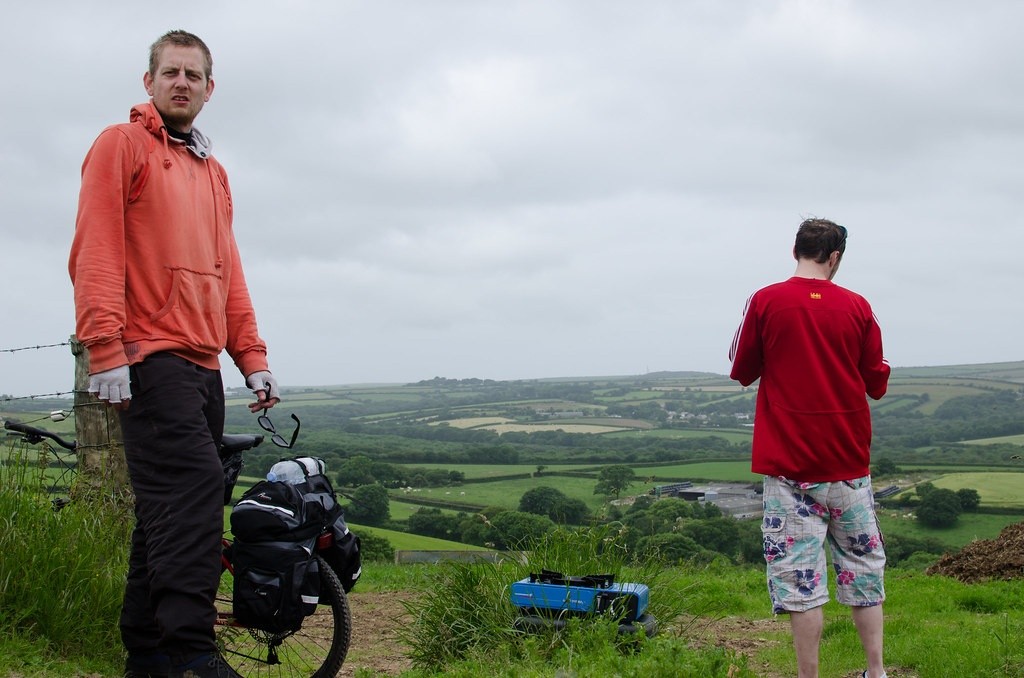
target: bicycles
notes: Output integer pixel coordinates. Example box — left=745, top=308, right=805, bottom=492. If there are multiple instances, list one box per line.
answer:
left=4, top=420, right=351, bottom=678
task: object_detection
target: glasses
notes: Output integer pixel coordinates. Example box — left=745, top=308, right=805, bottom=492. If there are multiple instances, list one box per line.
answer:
left=830, top=225, right=847, bottom=253
left=258, top=382, right=301, bottom=449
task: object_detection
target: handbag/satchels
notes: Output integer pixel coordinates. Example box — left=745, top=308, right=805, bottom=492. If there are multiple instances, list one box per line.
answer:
left=229, top=474, right=363, bottom=635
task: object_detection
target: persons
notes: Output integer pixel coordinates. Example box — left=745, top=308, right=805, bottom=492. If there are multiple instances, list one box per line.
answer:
left=69, top=29, right=281, bottom=678
left=729, top=218, right=890, bottom=677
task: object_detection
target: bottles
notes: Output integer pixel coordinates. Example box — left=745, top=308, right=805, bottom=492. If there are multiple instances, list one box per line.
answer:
left=266, top=456, right=326, bottom=486
